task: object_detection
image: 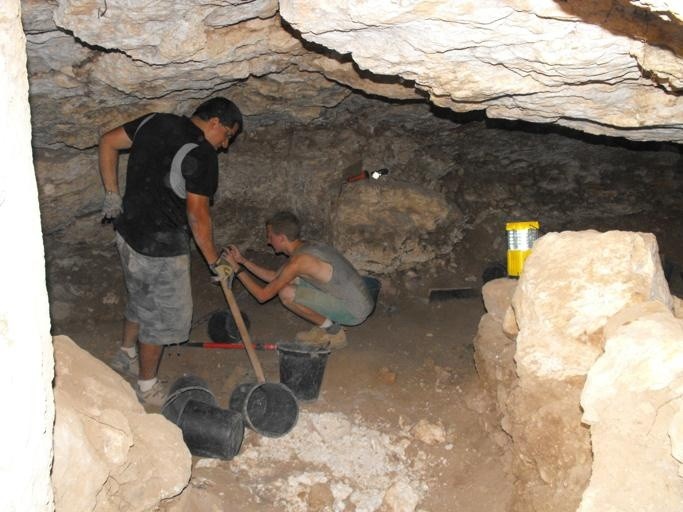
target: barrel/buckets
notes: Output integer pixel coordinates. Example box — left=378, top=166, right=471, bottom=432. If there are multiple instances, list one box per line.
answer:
left=159, top=374, right=218, bottom=425
left=277, top=342, right=331, bottom=403
left=506, top=220, right=543, bottom=277
left=228, top=382, right=300, bottom=437
left=176, top=397, right=245, bottom=460
left=208, top=308, right=250, bottom=343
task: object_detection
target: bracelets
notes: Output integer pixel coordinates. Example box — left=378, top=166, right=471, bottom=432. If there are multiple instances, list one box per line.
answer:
left=235, top=265, right=246, bottom=277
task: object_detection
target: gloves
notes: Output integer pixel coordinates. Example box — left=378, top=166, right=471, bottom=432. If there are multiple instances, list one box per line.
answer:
left=209, top=256, right=234, bottom=288
left=101, top=190, right=125, bottom=219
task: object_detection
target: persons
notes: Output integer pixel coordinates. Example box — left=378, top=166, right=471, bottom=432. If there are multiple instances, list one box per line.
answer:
left=221, top=211, right=375, bottom=351
left=99, top=97, right=243, bottom=407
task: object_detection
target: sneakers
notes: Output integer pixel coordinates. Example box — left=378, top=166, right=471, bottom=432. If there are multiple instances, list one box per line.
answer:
left=296, top=322, right=349, bottom=350
left=134, top=380, right=168, bottom=406
left=110, top=348, right=140, bottom=378
